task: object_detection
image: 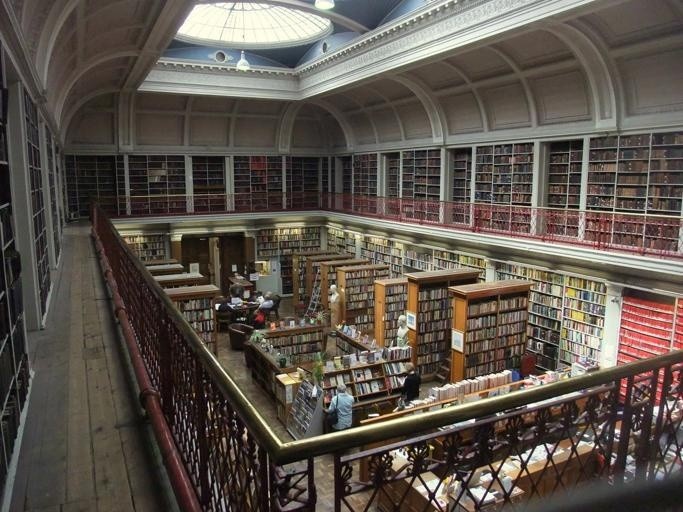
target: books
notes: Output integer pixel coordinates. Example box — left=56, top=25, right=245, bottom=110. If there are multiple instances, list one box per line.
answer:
left=0, top=244, right=31, bottom=492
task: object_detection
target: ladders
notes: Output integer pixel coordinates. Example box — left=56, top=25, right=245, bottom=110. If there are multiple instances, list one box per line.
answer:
left=433, top=349, right=452, bottom=387
left=306, top=270, right=323, bottom=314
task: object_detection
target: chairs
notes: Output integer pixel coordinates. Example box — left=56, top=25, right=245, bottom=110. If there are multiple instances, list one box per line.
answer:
left=216, top=312, right=231, bottom=332
left=261, top=295, right=282, bottom=323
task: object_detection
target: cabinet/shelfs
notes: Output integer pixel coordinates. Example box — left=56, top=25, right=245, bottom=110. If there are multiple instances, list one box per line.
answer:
left=248, top=337, right=324, bottom=440
left=246, top=230, right=358, bottom=300
left=123, top=230, right=221, bottom=360
left=228, top=276, right=253, bottom=298
left=293, top=235, right=608, bottom=391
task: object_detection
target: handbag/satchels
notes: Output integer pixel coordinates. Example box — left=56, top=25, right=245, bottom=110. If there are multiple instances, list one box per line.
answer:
left=327, top=412, right=338, bottom=426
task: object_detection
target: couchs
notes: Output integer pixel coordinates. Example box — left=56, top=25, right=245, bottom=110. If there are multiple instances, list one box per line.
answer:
left=228, top=323, right=254, bottom=350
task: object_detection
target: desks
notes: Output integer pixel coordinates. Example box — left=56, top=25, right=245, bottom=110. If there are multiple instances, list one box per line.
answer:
left=228, top=302, right=260, bottom=315
